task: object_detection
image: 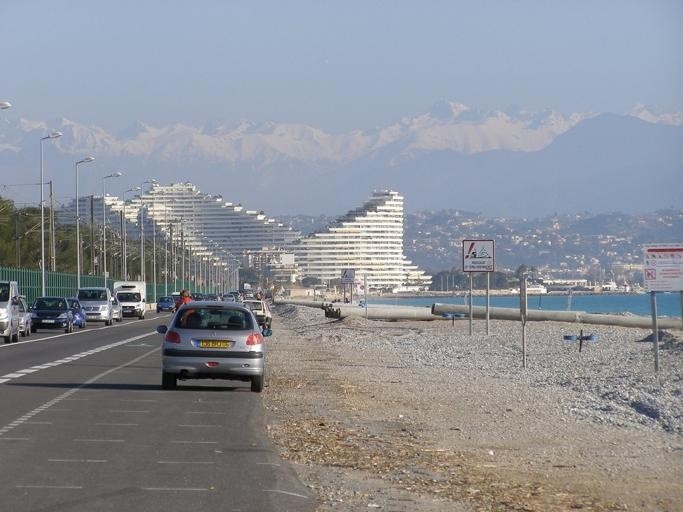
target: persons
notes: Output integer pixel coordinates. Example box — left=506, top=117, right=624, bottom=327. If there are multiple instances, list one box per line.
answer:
left=215, top=289, right=364, bottom=309
left=174, top=289, right=196, bottom=323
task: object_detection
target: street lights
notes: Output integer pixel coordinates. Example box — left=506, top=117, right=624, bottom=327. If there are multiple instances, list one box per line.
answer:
left=38, top=132, right=242, bottom=302
left=2, top=181, right=56, bottom=272
left=0, top=101, right=11, bottom=110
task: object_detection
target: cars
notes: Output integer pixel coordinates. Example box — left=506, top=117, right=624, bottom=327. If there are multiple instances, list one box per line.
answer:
left=0, top=280, right=273, bottom=345
left=156, top=300, right=272, bottom=394
